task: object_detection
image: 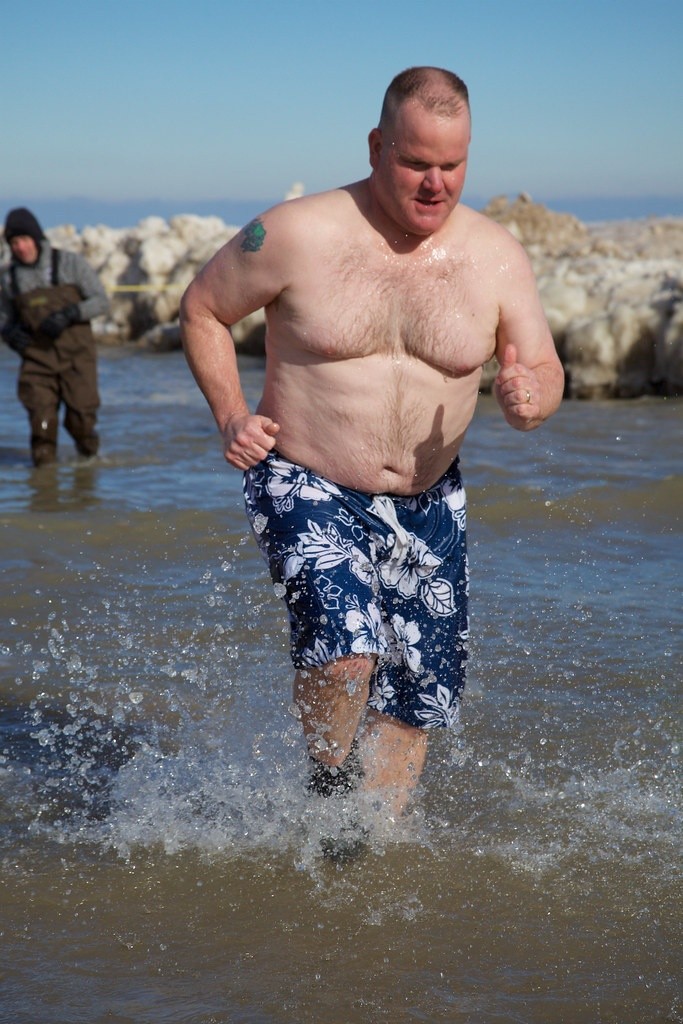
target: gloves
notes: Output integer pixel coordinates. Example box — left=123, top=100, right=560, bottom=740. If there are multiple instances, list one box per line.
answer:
left=3, top=317, right=35, bottom=355
left=35, top=303, right=80, bottom=350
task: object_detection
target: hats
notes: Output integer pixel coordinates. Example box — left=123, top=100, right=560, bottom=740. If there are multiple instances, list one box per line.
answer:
left=3, top=207, right=43, bottom=241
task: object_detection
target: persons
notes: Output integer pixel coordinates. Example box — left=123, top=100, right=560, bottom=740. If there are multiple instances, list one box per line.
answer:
left=176, top=62, right=566, bottom=859
left=1, top=207, right=110, bottom=470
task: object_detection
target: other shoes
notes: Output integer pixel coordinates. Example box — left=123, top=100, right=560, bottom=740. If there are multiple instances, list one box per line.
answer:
left=65, top=421, right=99, bottom=455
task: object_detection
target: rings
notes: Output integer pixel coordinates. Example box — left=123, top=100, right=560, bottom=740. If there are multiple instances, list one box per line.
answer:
left=527, top=389, right=532, bottom=403
